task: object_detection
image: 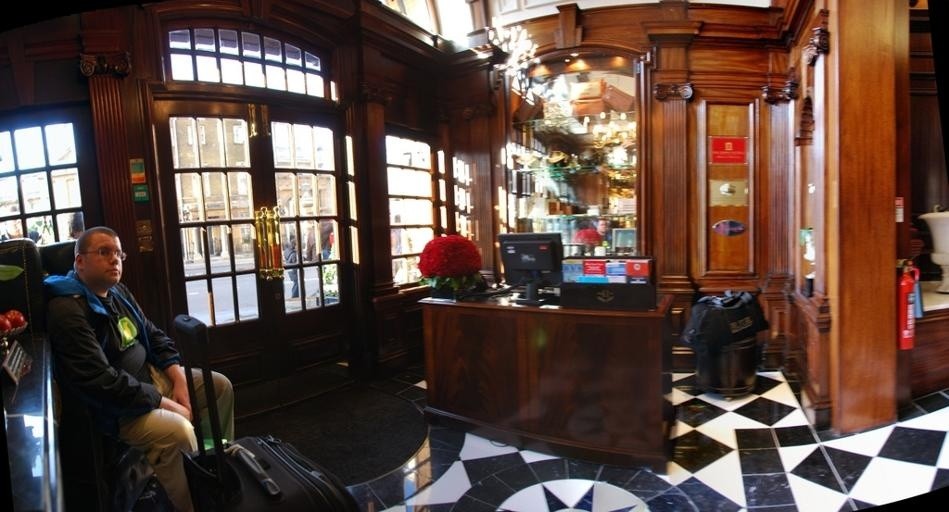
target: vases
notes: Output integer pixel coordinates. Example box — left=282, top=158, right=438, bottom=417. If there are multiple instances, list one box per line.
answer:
left=431, top=286, right=452, bottom=299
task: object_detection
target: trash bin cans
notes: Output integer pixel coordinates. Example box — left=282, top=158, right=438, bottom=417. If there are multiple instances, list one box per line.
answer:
left=693, top=294, right=759, bottom=398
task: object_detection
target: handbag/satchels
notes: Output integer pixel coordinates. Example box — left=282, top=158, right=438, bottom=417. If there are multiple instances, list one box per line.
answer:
left=680, top=288, right=770, bottom=348
left=107, top=436, right=173, bottom=512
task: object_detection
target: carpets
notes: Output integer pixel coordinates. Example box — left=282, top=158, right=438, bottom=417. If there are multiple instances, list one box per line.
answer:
left=234, top=383, right=427, bottom=489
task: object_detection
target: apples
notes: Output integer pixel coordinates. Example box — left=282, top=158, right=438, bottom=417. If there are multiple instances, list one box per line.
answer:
left=0, top=309, right=27, bottom=332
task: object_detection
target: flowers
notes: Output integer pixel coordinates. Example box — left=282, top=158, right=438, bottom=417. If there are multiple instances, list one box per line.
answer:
left=416, top=236, right=485, bottom=295
left=572, top=229, right=602, bottom=244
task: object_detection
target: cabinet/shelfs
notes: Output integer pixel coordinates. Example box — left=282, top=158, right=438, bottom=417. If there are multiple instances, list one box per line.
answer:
left=496, top=40, right=655, bottom=260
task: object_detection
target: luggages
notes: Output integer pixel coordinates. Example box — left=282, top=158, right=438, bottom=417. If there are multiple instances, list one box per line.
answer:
left=175, top=312, right=363, bottom=511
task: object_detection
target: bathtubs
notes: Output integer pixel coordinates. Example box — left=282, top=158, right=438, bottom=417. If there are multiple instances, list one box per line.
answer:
left=918, top=211, right=949, bottom=266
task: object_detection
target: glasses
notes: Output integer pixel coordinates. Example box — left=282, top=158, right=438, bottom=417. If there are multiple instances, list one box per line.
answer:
left=80, top=249, right=126, bottom=262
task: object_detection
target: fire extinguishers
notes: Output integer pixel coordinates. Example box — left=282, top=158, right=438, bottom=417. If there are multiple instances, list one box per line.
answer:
left=897, top=259, right=924, bottom=351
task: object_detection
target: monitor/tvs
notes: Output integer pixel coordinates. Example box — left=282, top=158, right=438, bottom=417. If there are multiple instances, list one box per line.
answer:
left=496, top=231, right=565, bottom=305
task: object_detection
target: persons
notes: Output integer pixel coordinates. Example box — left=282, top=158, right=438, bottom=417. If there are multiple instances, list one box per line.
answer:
left=574, top=219, right=609, bottom=246
left=284, top=214, right=333, bottom=299
left=0, top=205, right=84, bottom=244
left=45, top=225, right=235, bottom=512
